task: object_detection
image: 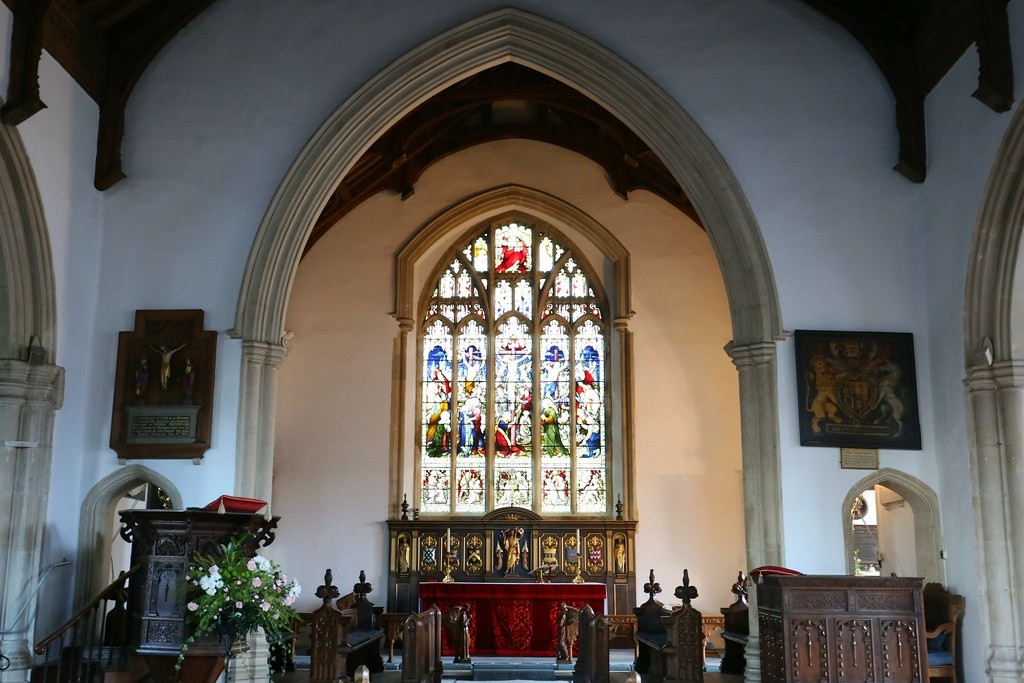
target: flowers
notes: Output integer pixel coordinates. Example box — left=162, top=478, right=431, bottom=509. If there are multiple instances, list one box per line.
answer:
left=175, top=533, right=302, bottom=683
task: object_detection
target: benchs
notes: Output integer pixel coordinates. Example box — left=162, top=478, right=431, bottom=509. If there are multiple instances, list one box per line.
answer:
left=923, top=581, right=962, bottom=683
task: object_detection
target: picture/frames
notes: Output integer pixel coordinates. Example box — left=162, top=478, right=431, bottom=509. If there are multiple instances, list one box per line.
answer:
left=794, top=330, right=922, bottom=450
left=111, top=308, right=218, bottom=458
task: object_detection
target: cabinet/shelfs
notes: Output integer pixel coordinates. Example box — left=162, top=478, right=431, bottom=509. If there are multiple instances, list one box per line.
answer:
left=419, top=582, right=607, bottom=658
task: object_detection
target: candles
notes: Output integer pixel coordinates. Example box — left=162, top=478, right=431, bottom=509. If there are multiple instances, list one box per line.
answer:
left=618, top=493, right=620, bottom=501
left=577, top=529, right=580, bottom=553
left=404, top=494, right=406, bottom=500
left=447, top=528, right=451, bottom=552
